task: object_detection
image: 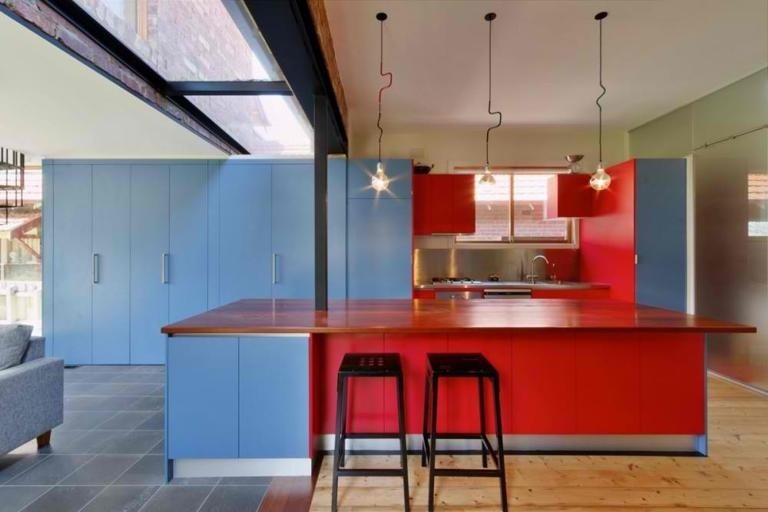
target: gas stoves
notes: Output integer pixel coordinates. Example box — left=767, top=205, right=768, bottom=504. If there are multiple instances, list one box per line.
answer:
left=428, top=277, right=481, bottom=286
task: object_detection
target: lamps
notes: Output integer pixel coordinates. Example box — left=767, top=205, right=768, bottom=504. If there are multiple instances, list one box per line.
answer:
left=588, top=14, right=613, bottom=190
left=477, top=13, right=502, bottom=192
left=369, top=13, right=392, bottom=190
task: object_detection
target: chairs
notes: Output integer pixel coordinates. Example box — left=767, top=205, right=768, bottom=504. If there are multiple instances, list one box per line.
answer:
left=1, top=337, right=63, bottom=458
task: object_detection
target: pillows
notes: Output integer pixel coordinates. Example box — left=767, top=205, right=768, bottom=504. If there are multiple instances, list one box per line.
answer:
left=1, top=325, right=34, bottom=372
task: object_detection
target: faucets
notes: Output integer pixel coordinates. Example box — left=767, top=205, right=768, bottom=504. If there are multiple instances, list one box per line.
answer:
left=526, top=255, right=550, bottom=282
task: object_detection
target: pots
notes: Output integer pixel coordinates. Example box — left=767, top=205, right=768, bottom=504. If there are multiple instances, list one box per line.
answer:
left=415, top=162, right=434, bottom=174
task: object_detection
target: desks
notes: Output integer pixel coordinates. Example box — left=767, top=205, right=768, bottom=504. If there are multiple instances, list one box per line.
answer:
left=160, top=298, right=758, bottom=334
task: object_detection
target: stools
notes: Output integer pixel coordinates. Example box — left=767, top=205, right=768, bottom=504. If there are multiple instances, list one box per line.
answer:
left=330, top=354, right=508, bottom=512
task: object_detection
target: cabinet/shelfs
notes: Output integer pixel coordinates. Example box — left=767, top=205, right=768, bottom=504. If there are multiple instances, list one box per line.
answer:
left=347, top=158, right=415, bottom=199
left=542, top=171, right=597, bottom=220
left=310, top=334, right=709, bottom=456
left=43, top=157, right=129, bottom=366
left=347, top=200, right=412, bottom=300
left=524, top=291, right=610, bottom=295
left=129, top=161, right=208, bottom=367
left=412, top=173, right=477, bottom=235
left=166, top=332, right=315, bottom=478
left=208, top=159, right=314, bottom=312
left=327, top=156, right=347, bottom=299
left=419, top=289, right=485, bottom=297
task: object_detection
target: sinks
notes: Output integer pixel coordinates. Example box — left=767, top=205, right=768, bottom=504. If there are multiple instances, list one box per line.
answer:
left=534, top=281, right=591, bottom=288
